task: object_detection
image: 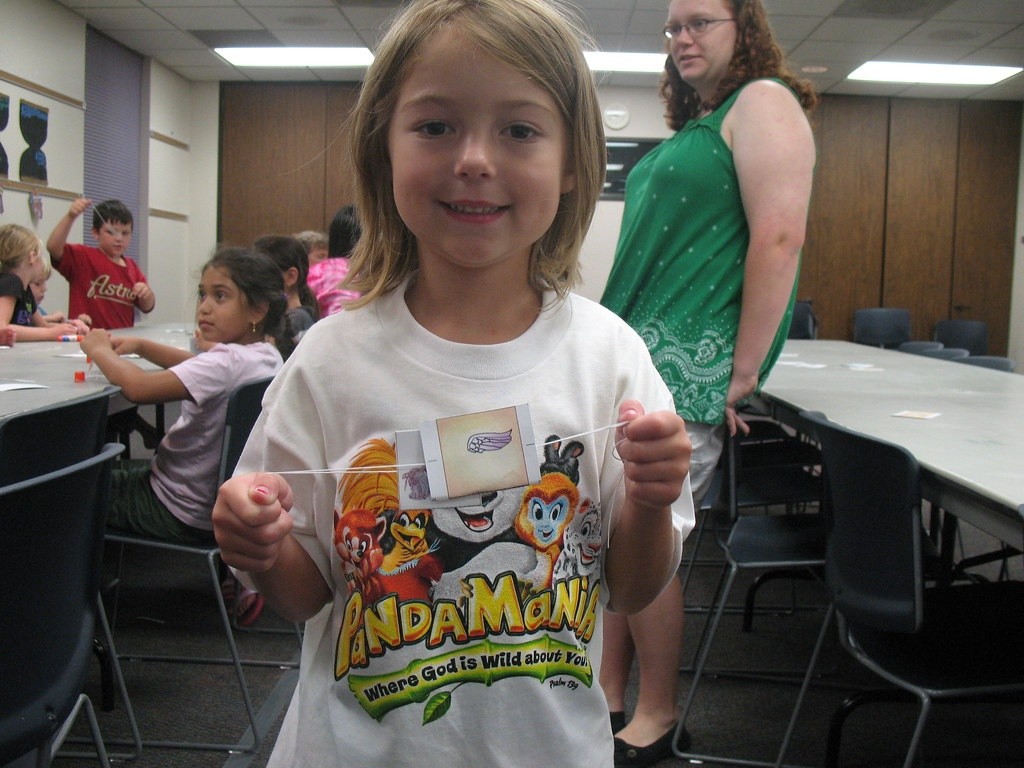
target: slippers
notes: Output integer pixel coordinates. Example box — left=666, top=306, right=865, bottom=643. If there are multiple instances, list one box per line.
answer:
left=220, top=576, right=263, bottom=627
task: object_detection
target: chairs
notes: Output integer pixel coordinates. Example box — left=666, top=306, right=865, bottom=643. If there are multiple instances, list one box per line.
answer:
left=0, top=361, right=292, bottom=768
left=675, top=305, right=1024, bottom=767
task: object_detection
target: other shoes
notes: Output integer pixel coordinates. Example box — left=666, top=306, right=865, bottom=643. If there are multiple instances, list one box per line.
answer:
left=614, top=721, right=691, bottom=768
left=608, top=711, right=627, bottom=736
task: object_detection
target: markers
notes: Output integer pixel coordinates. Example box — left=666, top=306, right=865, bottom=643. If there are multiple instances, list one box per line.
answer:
left=58, top=335, right=84, bottom=341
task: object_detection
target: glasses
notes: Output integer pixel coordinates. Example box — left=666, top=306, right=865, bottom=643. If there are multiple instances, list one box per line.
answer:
left=662, top=18, right=736, bottom=40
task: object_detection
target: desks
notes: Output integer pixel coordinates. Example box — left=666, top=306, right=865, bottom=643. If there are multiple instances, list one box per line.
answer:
left=756, top=338, right=1024, bottom=600
left=0, top=326, right=201, bottom=470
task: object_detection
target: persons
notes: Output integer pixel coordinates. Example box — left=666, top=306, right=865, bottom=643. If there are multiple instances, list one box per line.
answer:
left=306, top=203, right=384, bottom=324
left=0, top=225, right=92, bottom=347
left=293, top=230, right=328, bottom=266
left=80, top=248, right=282, bottom=618
left=212, top=0, right=695, bottom=768
left=196, top=235, right=317, bottom=627
left=588, top=0, right=817, bottom=768
left=47, top=196, right=154, bottom=333
